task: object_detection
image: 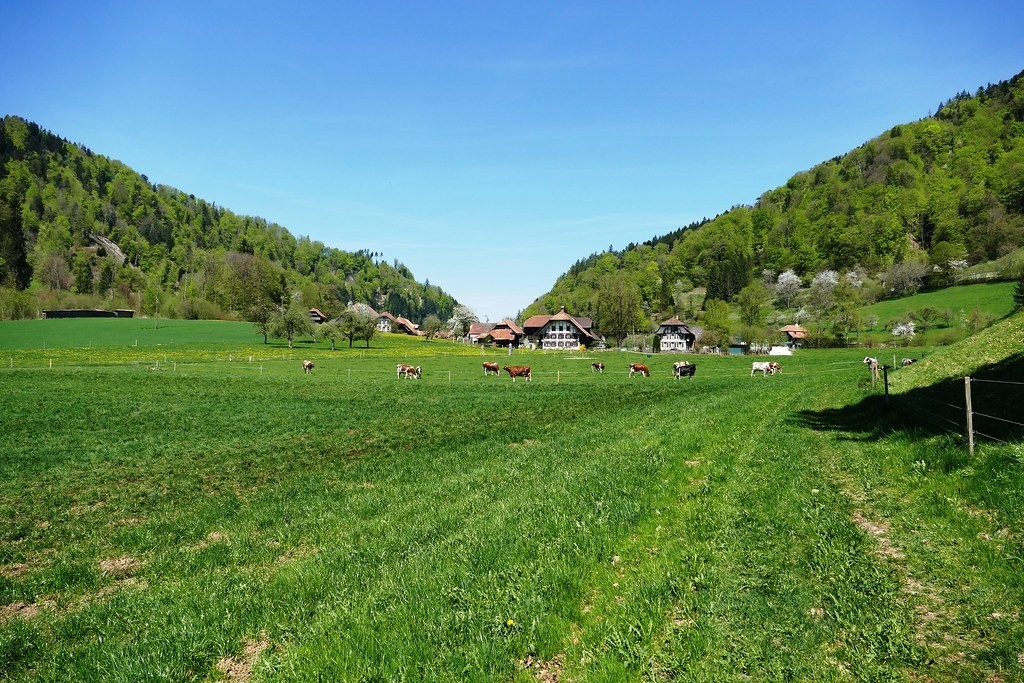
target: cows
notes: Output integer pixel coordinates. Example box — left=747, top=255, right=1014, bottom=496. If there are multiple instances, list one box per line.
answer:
left=672, top=364, right=698, bottom=381
left=482, top=362, right=501, bottom=377
left=672, top=361, right=690, bottom=374
left=628, top=362, right=651, bottom=378
left=901, top=358, right=913, bottom=368
left=415, top=366, right=423, bottom=378
left=502, top=365, right=532, bottom=382
left=767, top=360, right=783, bottom=374
left=302, top=360, right=314, bottom=374
left=750, top=361, right=771, bottom=378
left=396, top=363, right=417, bottom=380
left=863, top=356, right=878, bottom=371
left=592, top=362, right=605, bottom=374
